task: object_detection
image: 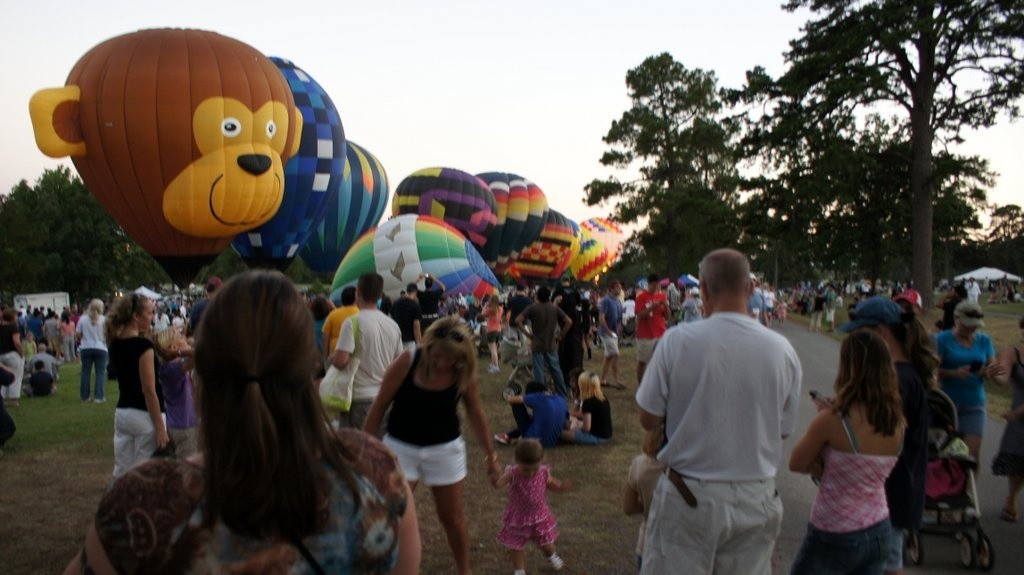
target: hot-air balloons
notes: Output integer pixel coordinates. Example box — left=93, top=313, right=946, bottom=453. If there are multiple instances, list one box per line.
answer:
left=469, top=172, right=551, bottom=276
left=388, top=165, right=499, bottom=259
left=495, top=209, right=583, bottom=299
left=299, top=139, right=388, bottom=291
left=328, top=213, right=503, bottom=332
left=231, top=58, right=346, bottom=274
left=556, top=218, right=630, bottom=292
left=27, top=27, right=303, bottom=306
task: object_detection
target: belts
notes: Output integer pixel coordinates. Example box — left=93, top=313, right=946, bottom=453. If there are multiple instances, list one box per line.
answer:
left=663, top=466, right=698, bottom=508
left=421, top=314, right=438, bottom=318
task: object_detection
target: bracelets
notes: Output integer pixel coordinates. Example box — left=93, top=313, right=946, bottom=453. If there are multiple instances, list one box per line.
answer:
left=484, top=455, right=498, bottom=464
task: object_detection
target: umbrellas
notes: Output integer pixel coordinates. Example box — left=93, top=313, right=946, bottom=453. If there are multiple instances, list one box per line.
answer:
left=133, top=285, right=162, bottom=301
left=954, top=266, right=1022, bottom=285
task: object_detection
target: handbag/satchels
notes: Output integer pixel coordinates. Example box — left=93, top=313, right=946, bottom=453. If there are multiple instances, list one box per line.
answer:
left=319, top=318, right=360, bottom=413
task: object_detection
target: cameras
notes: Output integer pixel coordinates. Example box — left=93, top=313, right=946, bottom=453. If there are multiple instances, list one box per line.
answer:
left=423, top=272, right=429, bottom=278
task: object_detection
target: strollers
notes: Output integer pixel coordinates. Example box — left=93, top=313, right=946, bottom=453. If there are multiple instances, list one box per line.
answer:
left=904, top=386, right=995, bottom=570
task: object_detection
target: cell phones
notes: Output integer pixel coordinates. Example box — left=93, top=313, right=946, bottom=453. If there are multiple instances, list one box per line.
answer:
left=809, top=389, right=823, bottom=399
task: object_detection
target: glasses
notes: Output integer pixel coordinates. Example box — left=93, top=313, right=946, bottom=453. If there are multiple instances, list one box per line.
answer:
left=434, top=328, right=464, bottom=342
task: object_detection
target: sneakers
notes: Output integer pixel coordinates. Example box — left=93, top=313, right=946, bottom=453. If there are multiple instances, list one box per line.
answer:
left=494, top=432, right=509, bottom=445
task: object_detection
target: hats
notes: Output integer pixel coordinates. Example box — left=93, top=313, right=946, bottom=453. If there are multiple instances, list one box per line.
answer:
left=749, top=273, right=757, bottom=281
left=135, top=286, right=161, bottom=299
left=836, top=296, right=901, bottom=334
left=893, top=290, right=922, bottom=311
left=954, top=301, right=984, bottom=328
left=207, top=277, right=223, bottom=288
left=691, top=287, right=699, bottom=295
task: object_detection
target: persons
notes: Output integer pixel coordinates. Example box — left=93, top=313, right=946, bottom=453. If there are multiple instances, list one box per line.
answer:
left=0, top=249, right=1024, bottom=575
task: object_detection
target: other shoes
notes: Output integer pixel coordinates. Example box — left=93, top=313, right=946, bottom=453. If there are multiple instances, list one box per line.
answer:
left=600, top=378, right=608, bottom=386
left=1003, top=498, right=1016, bottom=521
left=82, top=398, right=91, bottom=404
left=614, top=383, right=627, bottom=390
left=544, top=552, right=564, bottom=571
left=514, top=569, right=526, bottom=575
left=94, top=398, right=106, bottom=404
left=4, top=399, right=19, bottom=407
left=487, top=364, right=500, bottom=374
